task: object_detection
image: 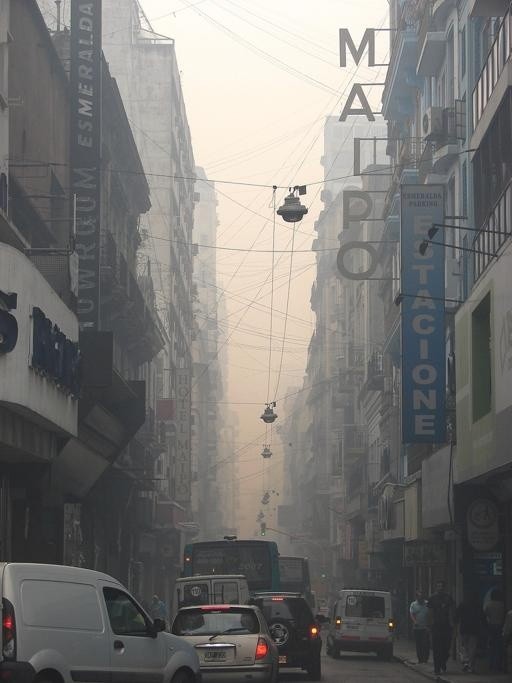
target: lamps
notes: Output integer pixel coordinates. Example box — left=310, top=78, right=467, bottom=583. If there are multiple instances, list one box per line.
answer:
left=261, top=403, right=278, bottom=423
left=277, top=187, right=308, bottom=222
left=393, top=218, right=510, bottom=306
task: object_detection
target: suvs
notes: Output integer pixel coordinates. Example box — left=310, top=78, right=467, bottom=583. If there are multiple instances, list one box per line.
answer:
left=171, top=536, right=326, bottom=681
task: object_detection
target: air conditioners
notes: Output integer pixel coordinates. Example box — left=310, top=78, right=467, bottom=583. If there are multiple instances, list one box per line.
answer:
left=420, top=104, right=443, bottom=140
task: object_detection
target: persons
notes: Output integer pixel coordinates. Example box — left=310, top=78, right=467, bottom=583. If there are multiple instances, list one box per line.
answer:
left=149, top=595, right=169, bottom=621
left=389, top=580, right=512, bottom=683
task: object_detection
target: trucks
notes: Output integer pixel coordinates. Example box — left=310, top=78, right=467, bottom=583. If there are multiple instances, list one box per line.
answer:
left=327, top=588, right=398, bottom=661
left=1, top=559, right=206, bottom=680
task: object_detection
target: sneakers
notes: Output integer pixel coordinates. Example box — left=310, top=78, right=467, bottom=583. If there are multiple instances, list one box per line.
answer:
left=419, top=658, right=505, bottom=676
